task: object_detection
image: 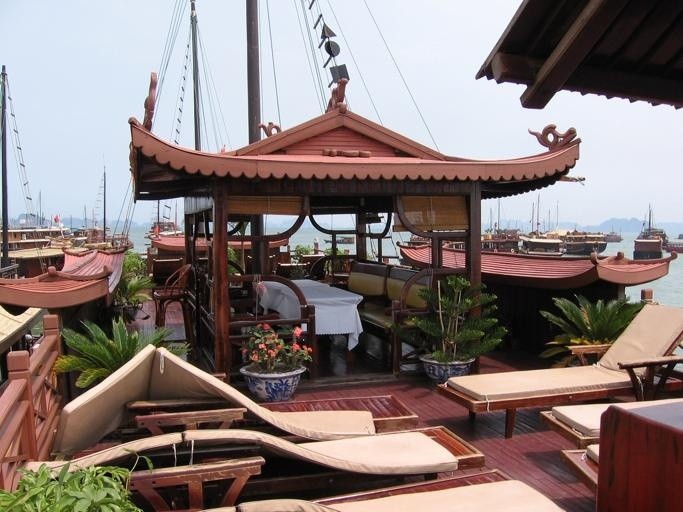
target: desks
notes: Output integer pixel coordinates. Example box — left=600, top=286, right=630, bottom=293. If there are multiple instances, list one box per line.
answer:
left=257, top=279, right=363, bottom=370
left=598, top=403, right=683, bottom=510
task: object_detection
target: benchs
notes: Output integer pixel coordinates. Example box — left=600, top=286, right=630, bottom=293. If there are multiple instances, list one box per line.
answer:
left=341, top=260, right=432, bottom=373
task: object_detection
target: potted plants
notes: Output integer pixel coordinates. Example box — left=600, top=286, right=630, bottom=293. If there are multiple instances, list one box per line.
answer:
left=239, top=325, right=313, bottom=404
left=382, top=274, right=510, bottom=390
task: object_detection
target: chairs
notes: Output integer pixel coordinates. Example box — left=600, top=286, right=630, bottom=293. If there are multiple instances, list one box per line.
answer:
left=436, top=302, right=682, bottom=438
left=27, top=425, right=484, bottom=511
left=213, top=470, right=563, bottom=510
left=540, top=398, right=683, bottom=448
left=153, top=264, right=198, bottom=360
left=562, top=444, right=599, bottom=491
left=56, top=345, right=418, bottom=455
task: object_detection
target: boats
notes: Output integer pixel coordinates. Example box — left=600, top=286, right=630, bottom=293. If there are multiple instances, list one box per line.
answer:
left=480, top=192, right=683, bottom=259
